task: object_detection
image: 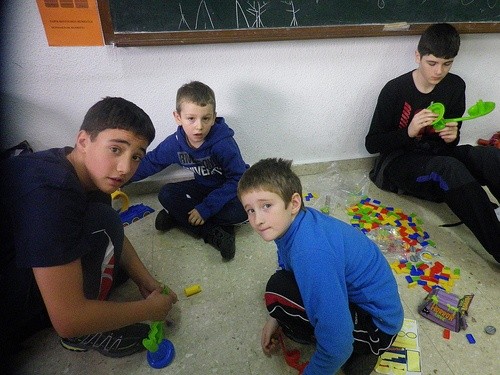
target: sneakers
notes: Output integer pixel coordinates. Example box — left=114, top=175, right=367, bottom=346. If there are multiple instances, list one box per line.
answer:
left=204, top=223, right=235, bottom=259
left=59, top=322, right=150, bottom=358
left=155, top=209, right=171, bottom=231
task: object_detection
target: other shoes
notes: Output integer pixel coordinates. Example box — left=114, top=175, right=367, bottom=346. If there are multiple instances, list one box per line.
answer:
left=344, top=352, right=378, bottom=375
left=281, top=327, right=311, bottom=345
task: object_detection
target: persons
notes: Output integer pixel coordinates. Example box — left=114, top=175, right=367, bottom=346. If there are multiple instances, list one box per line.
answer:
left=364, top=23, right=500, bottom=263
left=0, top=96, right=177, bottom=358
left=119, top=81, right=251, bottom=263
left=237, top=157, right=405, bottom=375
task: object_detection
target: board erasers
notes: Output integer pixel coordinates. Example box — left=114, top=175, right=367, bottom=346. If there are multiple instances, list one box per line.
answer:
left=383, top=21, right=411, bottom=32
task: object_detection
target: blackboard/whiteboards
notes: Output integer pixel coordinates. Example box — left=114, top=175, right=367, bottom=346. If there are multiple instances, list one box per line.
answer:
left=96, top=0, right=500, bottom=45
